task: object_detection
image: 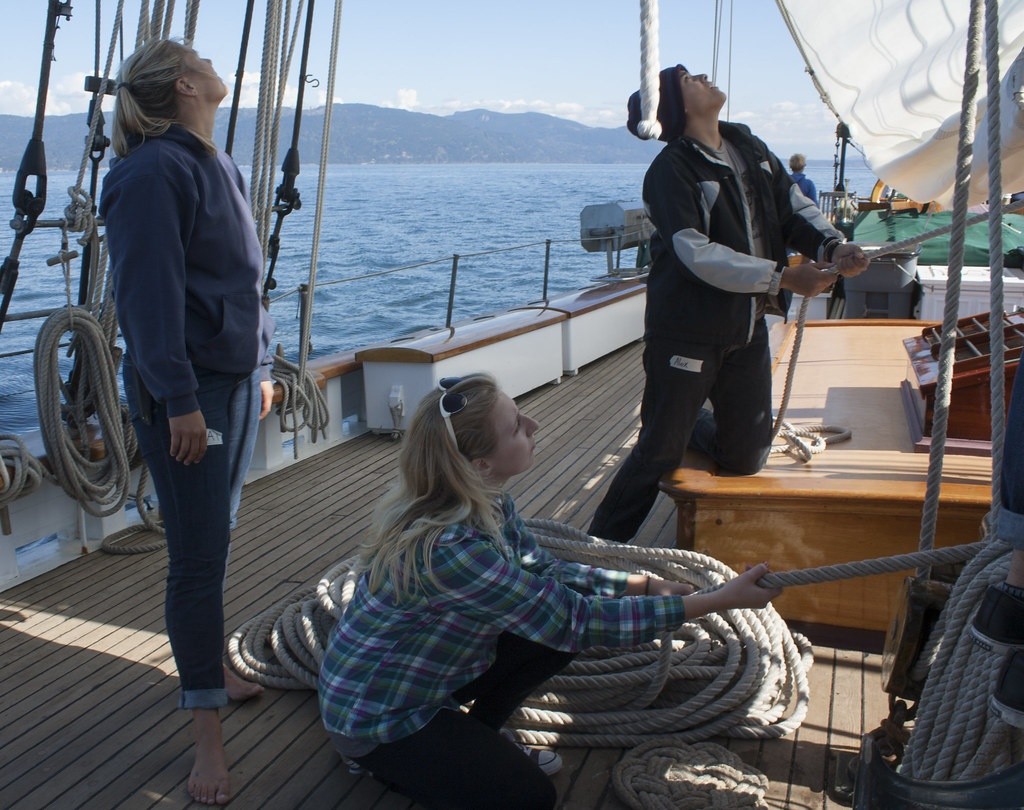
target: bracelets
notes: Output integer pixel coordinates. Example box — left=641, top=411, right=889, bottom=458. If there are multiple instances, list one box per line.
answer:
left=645, top=575, right=650, bottom=595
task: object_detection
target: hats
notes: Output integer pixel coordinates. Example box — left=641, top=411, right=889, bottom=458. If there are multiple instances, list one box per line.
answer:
left=626, top=63, right=688, bottom=141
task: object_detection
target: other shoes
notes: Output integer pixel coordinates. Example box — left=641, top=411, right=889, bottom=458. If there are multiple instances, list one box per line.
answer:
left=971, top=587, right=1024, bottom=654
left=990, top=648, right=1023, bottom=726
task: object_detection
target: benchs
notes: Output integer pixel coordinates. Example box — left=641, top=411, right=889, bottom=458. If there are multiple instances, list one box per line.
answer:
left=354, top=308, right=565, bottom=440
left=508, top=281, right=648, bottom=375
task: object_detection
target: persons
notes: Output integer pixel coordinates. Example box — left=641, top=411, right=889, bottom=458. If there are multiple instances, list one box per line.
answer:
left=586, top=63, right=871, bottom=545
left=98, top=39, right=277, bottom=805
left=789, top=154, right=817, bottom=203
left=318, top=370, right=783, bottom=810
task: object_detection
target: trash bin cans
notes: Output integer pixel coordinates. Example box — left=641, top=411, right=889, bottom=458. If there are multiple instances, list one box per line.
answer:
left=843, top=246, right=923, bottom=319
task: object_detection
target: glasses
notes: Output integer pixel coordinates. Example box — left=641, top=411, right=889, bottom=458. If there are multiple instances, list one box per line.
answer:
left=439, top=377, right=468, bottom=452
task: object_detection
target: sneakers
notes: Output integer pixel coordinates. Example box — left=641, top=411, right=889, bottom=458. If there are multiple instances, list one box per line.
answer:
left=513, top=742, right=562, bottom=776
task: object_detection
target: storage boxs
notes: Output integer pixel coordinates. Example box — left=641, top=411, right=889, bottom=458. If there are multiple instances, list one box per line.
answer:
left=915, top=263, right=1024, bottom=322
